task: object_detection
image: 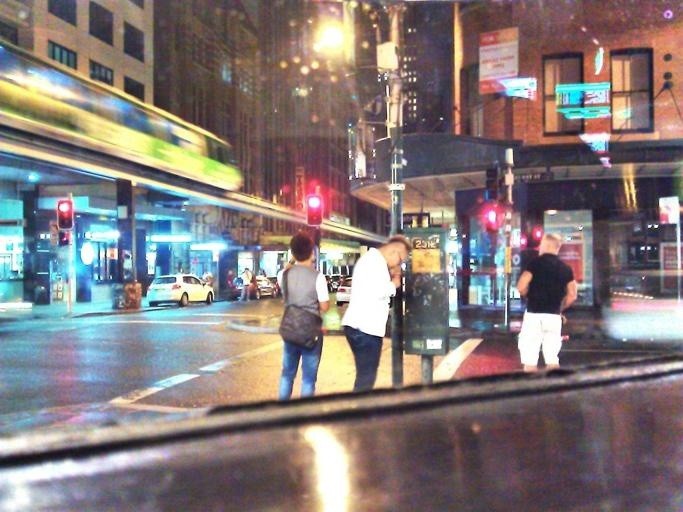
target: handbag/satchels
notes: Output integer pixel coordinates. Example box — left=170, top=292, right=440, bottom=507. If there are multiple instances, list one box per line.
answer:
left=279, top=304, right=322, bottom=348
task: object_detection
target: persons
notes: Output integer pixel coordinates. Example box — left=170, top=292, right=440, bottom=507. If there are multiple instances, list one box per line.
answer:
left=513, top=233, right=579, bottom=372
left=274, top=231, right=331, bottom=403
left=238, top=267, right=251, bottom=302
left=341, top=234, right=412, bottom=393
left=202, top=269, right=213, bottom=283
left=227, top=269, right=238, bottom=300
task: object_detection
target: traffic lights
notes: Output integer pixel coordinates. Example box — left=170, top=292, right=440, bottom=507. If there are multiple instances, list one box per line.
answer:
left=475, top=200, right=506, bottom=233
left=306, top=191, right=323, bottom=228
left=56, top=199, right=75, bottom=232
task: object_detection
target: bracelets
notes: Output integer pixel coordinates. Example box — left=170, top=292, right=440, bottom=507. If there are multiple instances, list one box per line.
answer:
left=288, top=259, right=294, bottom=265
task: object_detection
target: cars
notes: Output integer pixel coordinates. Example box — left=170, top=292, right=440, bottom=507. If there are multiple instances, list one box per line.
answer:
left=230, top=275, right=277, bottom=300
left=146, top=272, right=215, bottom=307
left=334, top=277, right=352, bottom=307
